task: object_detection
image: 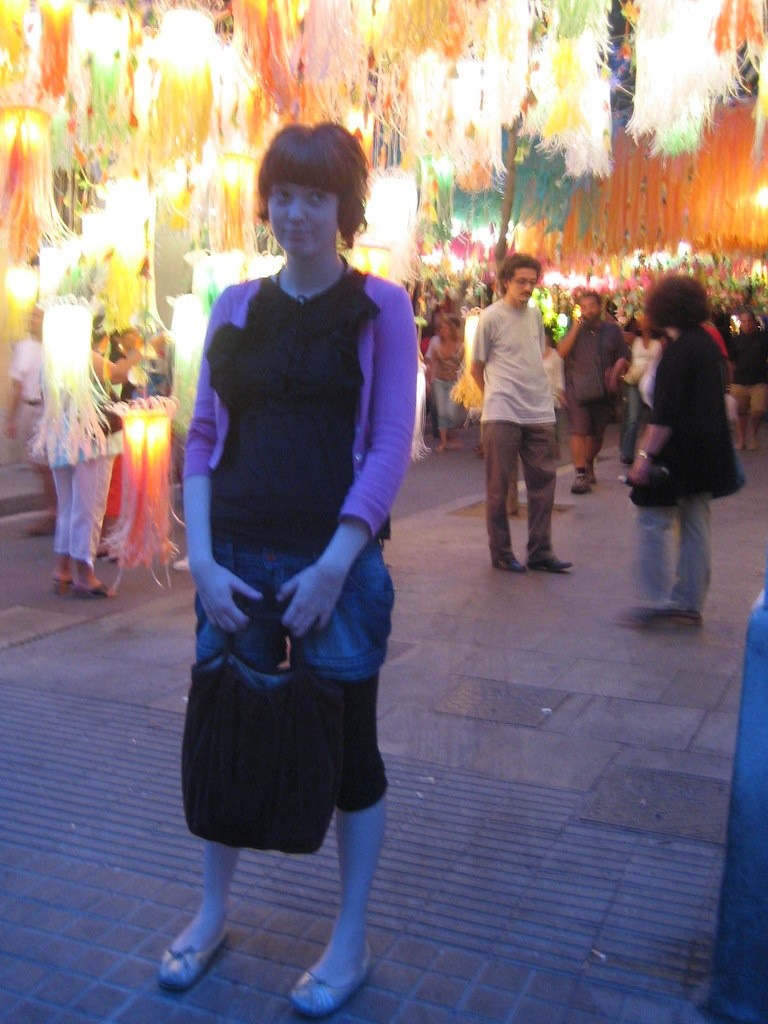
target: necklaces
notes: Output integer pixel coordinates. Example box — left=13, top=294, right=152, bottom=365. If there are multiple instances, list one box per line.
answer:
left=277, top=253, right=348, bottom=307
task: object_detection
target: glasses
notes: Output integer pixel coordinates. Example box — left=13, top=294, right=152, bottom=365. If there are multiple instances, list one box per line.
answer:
left=507, top=275, right=536, bottom=287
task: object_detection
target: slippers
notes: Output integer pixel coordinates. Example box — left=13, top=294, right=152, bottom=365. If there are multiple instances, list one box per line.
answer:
left=73, top=581, right=119, bottom=599
left=50, top=572, right=73, bottom=594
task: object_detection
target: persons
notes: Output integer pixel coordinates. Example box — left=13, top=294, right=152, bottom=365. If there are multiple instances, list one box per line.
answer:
left=154, top=123, right=419, bottom=1011
left=613, top=276, right=746, bottom=633
left=418, top=283, right=766, bottom=455
left=470, top=251, right=573, bottom=573
left=3, top=301, right=172, bottom=601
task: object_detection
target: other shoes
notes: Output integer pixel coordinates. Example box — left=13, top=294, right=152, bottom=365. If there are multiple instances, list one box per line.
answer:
left=735, top=441, right=747, bottom=450
left=620, top=453, right=633, bottom=464
left=27, top=514, right=56, bottom=534
left=288, top=938, right=373, bottom=1017
left=571, top=473, right=590, bottom=493
left=586, top=462, right=595, bottom=482
left=748, top=437, right=759, bottom=450
left=159, top=924, right=230, bottom=989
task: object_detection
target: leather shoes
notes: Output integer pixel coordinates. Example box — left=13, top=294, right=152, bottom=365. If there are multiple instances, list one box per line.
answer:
left=492, top=556, right=526, bottom=572
left=528, top=556, right=574, bottom=571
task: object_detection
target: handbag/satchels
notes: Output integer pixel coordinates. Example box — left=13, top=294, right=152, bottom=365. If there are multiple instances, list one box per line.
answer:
left=179, top=600, right=348, bottom=854
